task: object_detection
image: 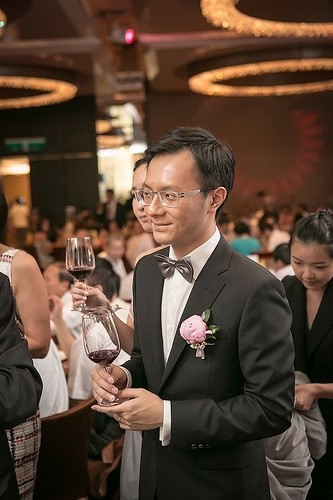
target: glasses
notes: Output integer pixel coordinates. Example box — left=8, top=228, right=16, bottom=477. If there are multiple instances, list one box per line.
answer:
left=134, top=187, right=216, bottom=208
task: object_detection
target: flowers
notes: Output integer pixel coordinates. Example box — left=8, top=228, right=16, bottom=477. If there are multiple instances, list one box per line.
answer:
left=179, top=309, right=222, bottom=359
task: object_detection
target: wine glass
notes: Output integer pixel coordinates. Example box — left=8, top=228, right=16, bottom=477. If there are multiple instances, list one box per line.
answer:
left=66, top=236, right=96, bottom=312
left=82, top=309, right=121, bottom=408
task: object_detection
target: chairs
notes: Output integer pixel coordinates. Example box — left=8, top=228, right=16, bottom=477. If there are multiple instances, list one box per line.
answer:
left=33, top=397, right=98, bottom=500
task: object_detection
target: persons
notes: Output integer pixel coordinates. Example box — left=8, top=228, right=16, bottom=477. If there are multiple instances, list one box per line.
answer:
left=0, top=271, right=42, bottom=500
left=42, top=260, right=90, bottom=342
left=90, top=127, right=296, bottom=500
left=1, top=183, right=333, bottom=308
left=70, top=156, right=170, bottom=500
left=30, top=336, right=68, bottom=420
left=47, top=256, right=132, bottom=407
left=0, top=187, right=51, bottom=499
left=279, top=208, right=332, bottom=499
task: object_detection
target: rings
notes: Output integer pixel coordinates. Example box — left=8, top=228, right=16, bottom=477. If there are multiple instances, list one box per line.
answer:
left=117, top=416, right=122, bottom=423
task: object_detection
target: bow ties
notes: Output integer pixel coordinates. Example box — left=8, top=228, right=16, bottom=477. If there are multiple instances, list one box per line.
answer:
left=151, top=252, right=193, bottom=284
